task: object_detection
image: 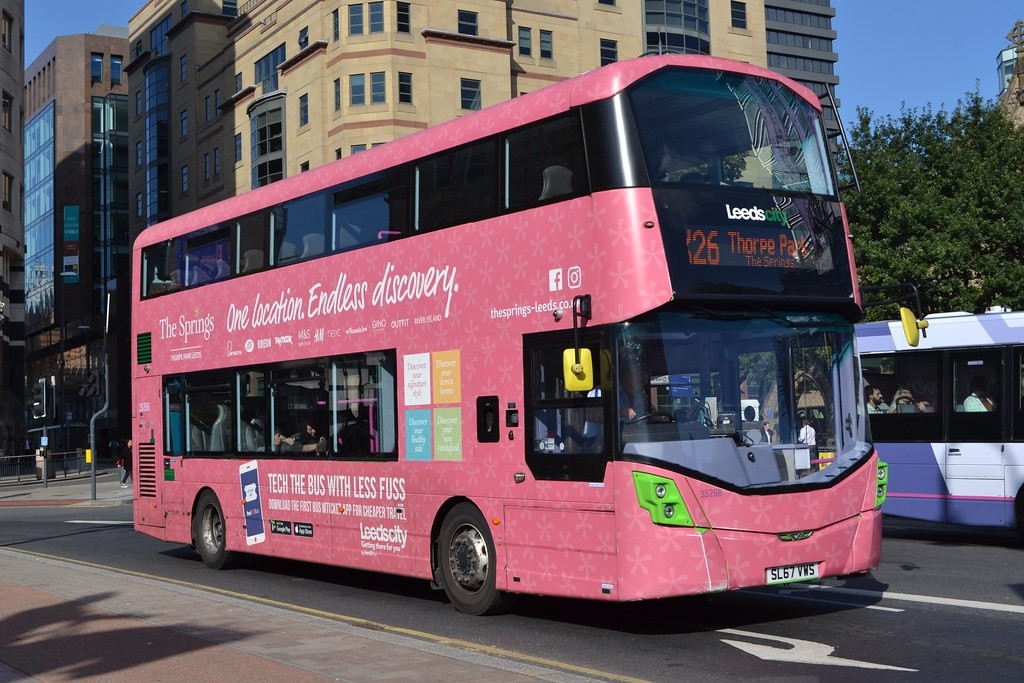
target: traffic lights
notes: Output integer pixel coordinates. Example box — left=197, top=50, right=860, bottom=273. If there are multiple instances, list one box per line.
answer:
left=77, top=370, right=101, bottom=396
left=32, top=378, right=46, bottom=419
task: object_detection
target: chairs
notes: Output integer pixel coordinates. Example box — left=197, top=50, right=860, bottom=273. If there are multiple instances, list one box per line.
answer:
left=895, top=396, right=935, bottom=413
left=166, top=225, right=362, bottom=291
left=181, top=400, right=266, bottom=451
left=537, top=165, right=575, bottom=201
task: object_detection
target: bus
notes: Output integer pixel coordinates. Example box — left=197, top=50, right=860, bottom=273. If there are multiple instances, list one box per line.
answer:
left=131, top=54, right=928, bottom=616
left=854, top=304, right=1024, bottom=545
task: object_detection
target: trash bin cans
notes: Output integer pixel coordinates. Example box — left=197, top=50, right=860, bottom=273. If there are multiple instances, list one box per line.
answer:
left=46, top=460, right=56, bottom=479
left=120, top=465, right=132, bottom=483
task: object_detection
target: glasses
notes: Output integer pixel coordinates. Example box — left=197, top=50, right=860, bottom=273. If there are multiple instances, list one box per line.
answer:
left=901, top=391, right=911, bottom=395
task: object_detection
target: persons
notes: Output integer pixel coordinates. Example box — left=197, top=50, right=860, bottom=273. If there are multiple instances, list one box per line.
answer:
left=583, top=358, right=658, bottom=437
left=22, top=432, right=35, bottom=469
left=963, top=374, right=997, bottom=411
left=120, top=440, right=132, bottom=488
left=865, top=383, right=930, bottom=414
left=760, top=421, right=775, bottom=443
left=274, top=423, right=327, bottom=452
left=798, top=420, right=819, bottom=472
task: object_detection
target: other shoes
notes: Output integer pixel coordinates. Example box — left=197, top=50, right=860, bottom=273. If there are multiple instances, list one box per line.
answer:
left=121, top=483, right=128, bottom=489
